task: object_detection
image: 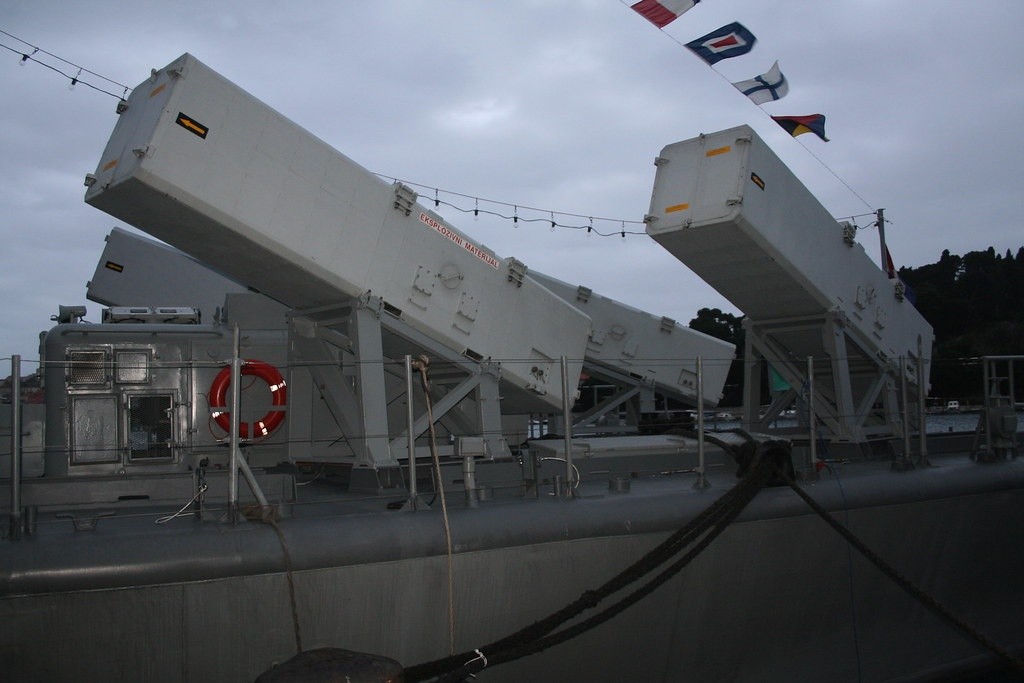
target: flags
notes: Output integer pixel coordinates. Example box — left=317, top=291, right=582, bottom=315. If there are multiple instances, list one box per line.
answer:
left=683, top=22, right=757, bottom=67
left=732, top=60, right=790, bottom=105
left=630, top=0, right=700, bottom=30
left=771, top=113, right=831, bottom=142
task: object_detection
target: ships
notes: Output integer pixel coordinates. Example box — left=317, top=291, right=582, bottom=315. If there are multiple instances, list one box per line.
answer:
left=0, top=0, right=1024, bottom=683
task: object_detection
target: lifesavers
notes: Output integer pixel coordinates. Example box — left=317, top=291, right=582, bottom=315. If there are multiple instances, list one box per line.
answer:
left=205, top=358, right=289, bottom=440
